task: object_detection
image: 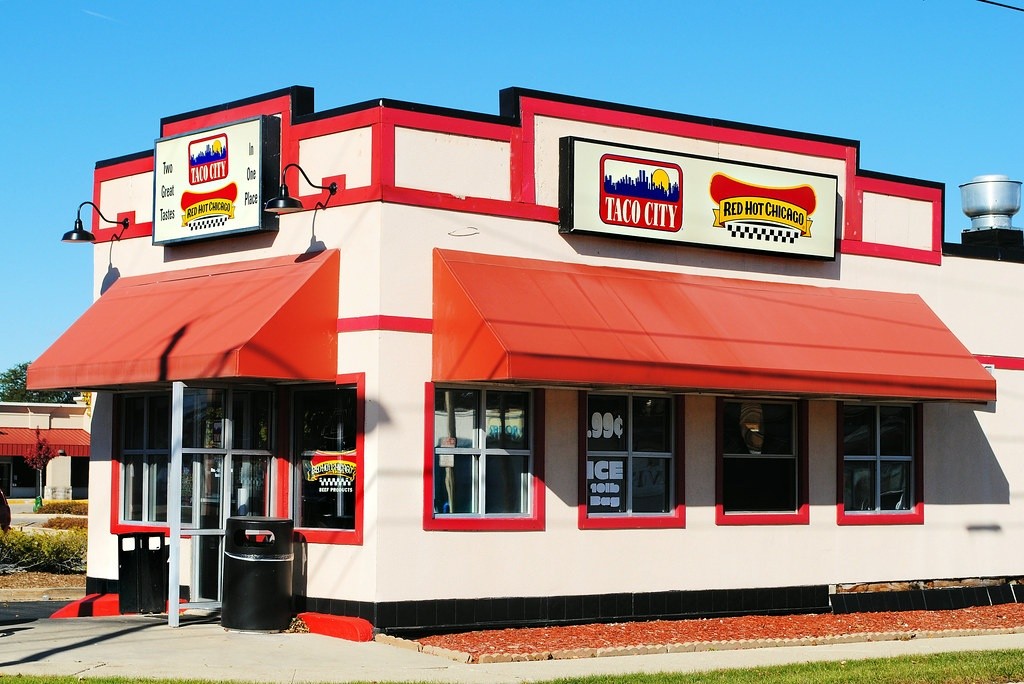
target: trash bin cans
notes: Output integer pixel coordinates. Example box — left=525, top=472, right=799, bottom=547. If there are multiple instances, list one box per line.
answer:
left=117, top=532, right=167, bottom=613
left=221, top=516, right=295, bottom=632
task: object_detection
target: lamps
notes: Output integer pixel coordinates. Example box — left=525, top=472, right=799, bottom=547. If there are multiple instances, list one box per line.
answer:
left=61, top=201, right=130, bottom=243
left=263, top=162, right=338, bottom=213
